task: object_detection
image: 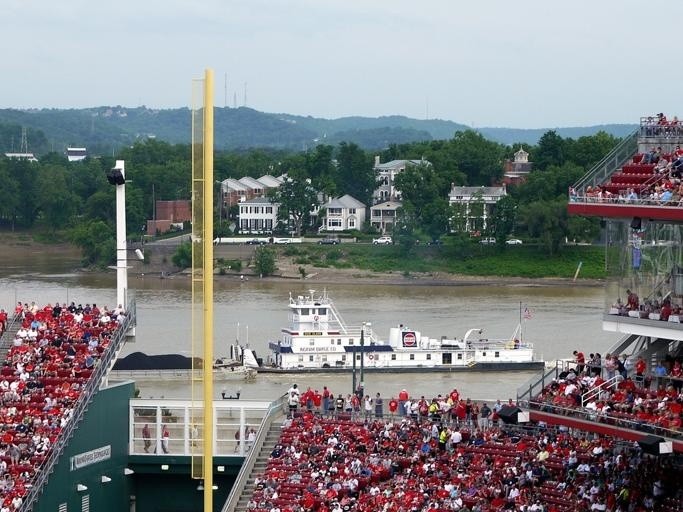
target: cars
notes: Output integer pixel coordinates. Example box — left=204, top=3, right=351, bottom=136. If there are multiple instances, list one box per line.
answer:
left=274, top=239, right=290, bottom=245
left=425, top=240, right=443, bottom=245
left=246, top=239, right=265, bottom=245
left=504, top=239, right=521, bottom=245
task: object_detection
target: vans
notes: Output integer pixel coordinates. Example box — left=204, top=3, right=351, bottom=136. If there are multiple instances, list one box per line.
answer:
left=478, top=237, right=496, bottom=245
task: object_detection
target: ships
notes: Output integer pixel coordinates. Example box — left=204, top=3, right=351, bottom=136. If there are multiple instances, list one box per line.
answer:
left=211, top=287, right=544, bottom=374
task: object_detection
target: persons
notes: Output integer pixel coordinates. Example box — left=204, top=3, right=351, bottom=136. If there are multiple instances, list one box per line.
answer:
left=585, top=112, right=682, bottom=207
left=1, top=300, right=127, bottom=512
left=142, top=423, right=152, bottom=453
left=474, top=230, right=480, bottom=236
left=189, top=424, right=198, bottom=446
left=513, top=339, right=520, bottom=349
left=153, top=424, right=170, bottom=454
left=233, top=350, right=682, bottom=511
left=615, top=287, right=682, bottom=324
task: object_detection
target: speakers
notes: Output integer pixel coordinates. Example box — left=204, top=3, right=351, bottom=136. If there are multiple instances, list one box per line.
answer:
left=106, top=168, right=125, bottom=185
left=639, top=435, right=667, bottom=457
left=498, top=405, right=523, bottom=425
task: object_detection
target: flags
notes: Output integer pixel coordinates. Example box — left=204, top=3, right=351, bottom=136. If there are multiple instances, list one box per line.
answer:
left=522, top=307, right=534, bottom=319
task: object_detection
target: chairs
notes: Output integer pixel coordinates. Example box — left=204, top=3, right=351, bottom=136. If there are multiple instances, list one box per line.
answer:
left=578, top=117, right=682, bottom=207
left=0, top=305, right=126, bottom=512
left=610, top=303, right=683, bottom=326
left=246, top=364, right=683, bottom=511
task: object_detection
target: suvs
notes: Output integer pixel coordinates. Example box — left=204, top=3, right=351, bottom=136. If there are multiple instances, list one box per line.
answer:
left=372, top=237, right=392, bottom=245
left=316, top=235, right=340, bottom=245
